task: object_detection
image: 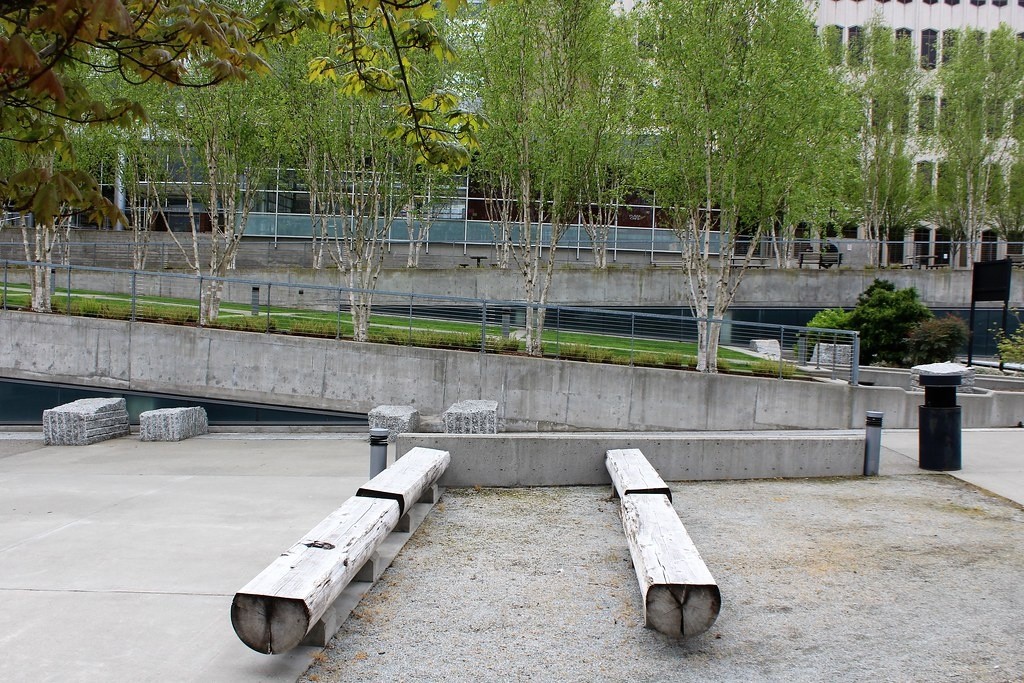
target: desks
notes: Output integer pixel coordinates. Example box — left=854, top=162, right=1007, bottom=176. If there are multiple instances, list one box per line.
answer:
left=470, top=256, right=488, bottom=268
left=728, top=257, right=769, bottom=270
left=905, top=254, right=939, bottom=270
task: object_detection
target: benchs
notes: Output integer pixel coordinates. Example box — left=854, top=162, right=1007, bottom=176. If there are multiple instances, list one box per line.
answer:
left=619, top=494, right=722, bottom=643
left=900, top=264, right=913, bottom=269
left=604, top=447, right=673, bottom=505
left=650, top=261, right=682, bottom=269
left=796, top=252, right=844, bottom=270
left=927, top=265, right=944, bottom=270
left=230, top=495, right=402, bottom=655
left=727, top=264, right=771, bottom=270
left=355, top=445, right=452, bottom=533
left=488, top=263, right=497, bottom=268
left=460, top=264, right=469, bottom=268
left=1004, top=254, right=1024, bottom=269
